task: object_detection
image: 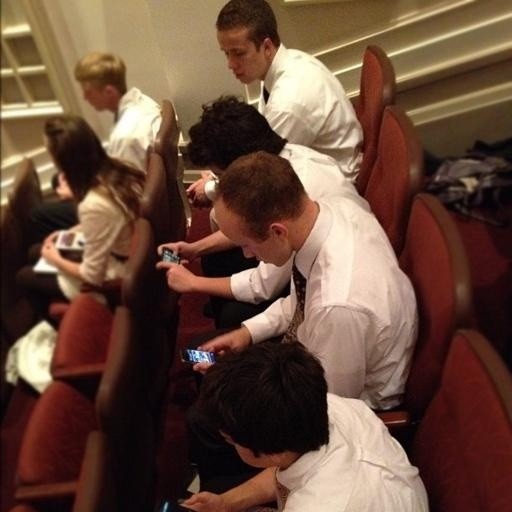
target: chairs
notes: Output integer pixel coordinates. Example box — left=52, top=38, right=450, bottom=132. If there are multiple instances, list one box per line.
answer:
left=352, top=44, right=511, bottom=511
left=1, top=96, right=190, bottom=512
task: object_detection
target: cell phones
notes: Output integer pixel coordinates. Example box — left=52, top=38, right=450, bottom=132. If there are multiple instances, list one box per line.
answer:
left=162, top=246, right=180, bottom=265
left=161, top=501, right=197, bottom=512
left=180, top=347, right=218, bottom=365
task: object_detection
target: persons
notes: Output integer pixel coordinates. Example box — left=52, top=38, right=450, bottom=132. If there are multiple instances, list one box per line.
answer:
left=177, top=0, right=365, bottom=234
left=145, top=93, right=372, bottom=331
left=176, top=148, right=421, bottom=495
left=179, top=332, right=433, bottom=512
left=15, top=50, right=185, bottom=263
left=9, top=111, right=147, bottom=320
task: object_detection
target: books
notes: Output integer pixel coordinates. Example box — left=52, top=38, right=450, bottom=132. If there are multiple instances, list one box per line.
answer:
left=30, top=229, right=85, bottom=272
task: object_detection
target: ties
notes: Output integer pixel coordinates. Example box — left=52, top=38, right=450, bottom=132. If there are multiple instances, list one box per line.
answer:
left=280, top=263, right=307, bottom=350
